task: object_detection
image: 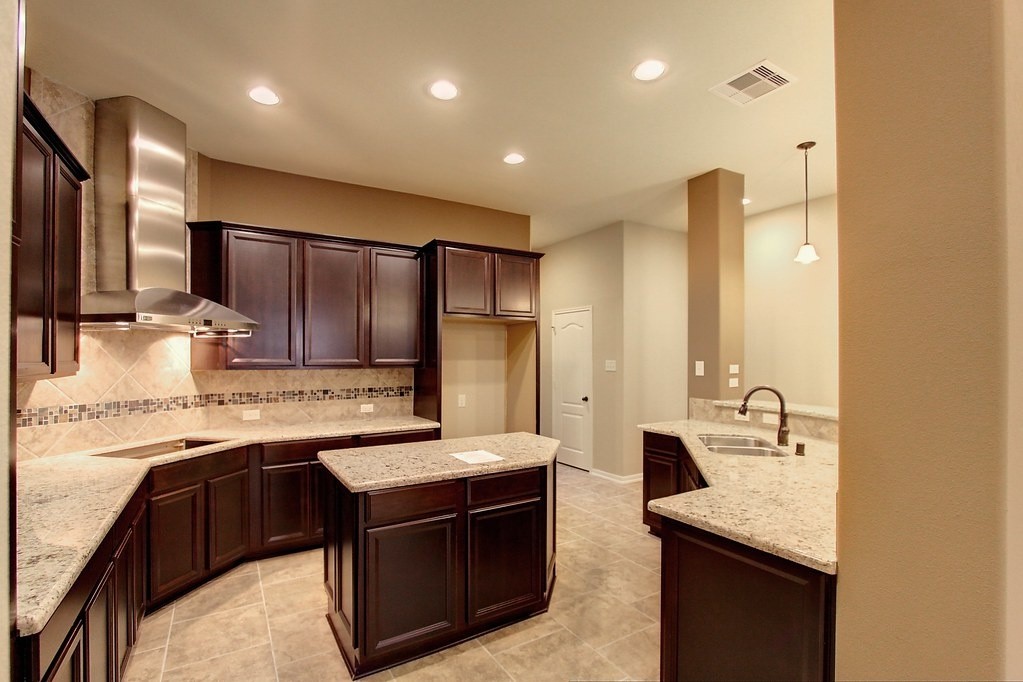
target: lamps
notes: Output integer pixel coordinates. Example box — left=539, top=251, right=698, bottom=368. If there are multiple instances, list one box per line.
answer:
left=791, top=142, right=818, bottom=263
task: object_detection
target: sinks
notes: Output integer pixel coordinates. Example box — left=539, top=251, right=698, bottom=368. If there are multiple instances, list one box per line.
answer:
left=706, top=446, right=790, bottom=458
left=697, top=433, right=775, bottom=447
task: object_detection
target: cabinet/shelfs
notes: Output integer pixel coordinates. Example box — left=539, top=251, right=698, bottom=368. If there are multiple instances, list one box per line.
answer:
left=17, top=89, right=92, bottom=385
left=677, top=436, right=709, bottom=494
left=151, top=446, right=250, bottom=609
left=546, top=454, right=556, bottom=610
left=660, top=515, right=835, bottom=682
left=358, top=428, right=435, bottom=446
left=13, top=470, right=149, bottom=682
left=413, top=241, right=542, bottom=439
left=323, top=468, right=355, bottom=681
left=186, top=220, right=422, bottom=369
left=250, top=436, right=353, bottom=559
left=355, top=465, right=546, bottom=678
left=643, top=431, right=677, bottom=536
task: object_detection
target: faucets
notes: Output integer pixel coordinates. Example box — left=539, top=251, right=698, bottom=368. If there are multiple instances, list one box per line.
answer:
left=737, top=384, right=790, bottom=447
